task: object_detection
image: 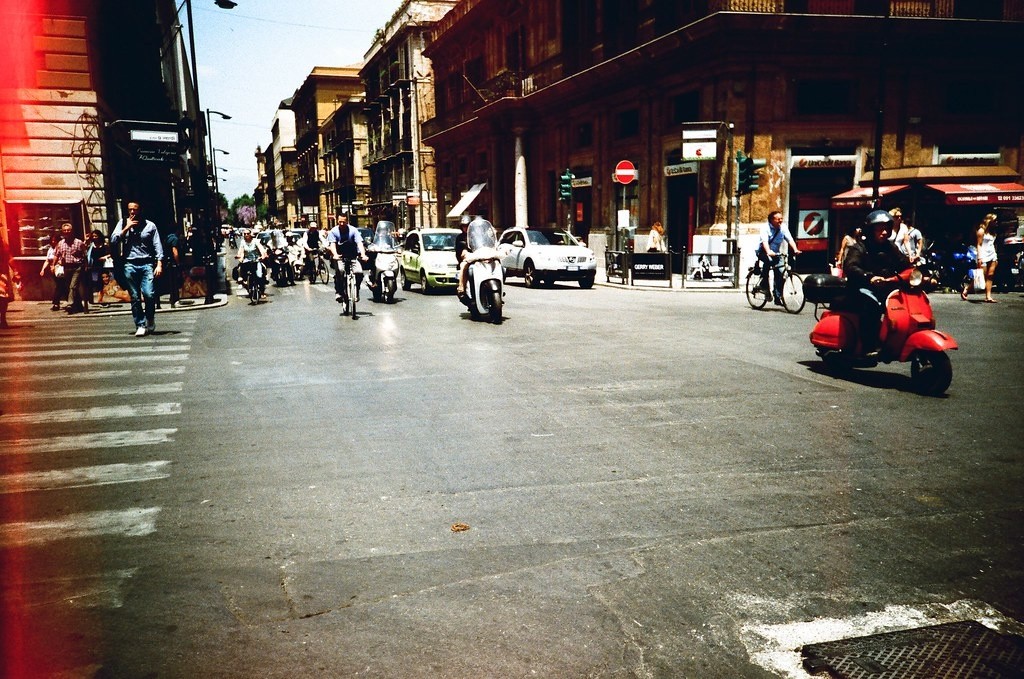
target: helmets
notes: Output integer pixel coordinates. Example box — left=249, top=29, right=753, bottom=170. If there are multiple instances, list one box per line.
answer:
left=863, top=210, right=895, bottom=238
left=459, top=216, right=471, bottom=231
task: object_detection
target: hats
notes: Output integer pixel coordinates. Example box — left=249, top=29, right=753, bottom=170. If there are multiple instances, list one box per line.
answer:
left=310, top=222, right=317, bottom=228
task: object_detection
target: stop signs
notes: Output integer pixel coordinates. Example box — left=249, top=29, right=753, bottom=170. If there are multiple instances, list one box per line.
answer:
left=614, top=159, right=635, bottom=185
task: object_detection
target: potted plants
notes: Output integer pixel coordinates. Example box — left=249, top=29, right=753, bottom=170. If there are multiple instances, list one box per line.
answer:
left=498, top=70, right=518, bottom=98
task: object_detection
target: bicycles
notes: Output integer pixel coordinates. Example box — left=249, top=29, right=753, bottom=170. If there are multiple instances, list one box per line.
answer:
left=746, top=253, right=805, bottom=313
left=291, top=244, right=330, bottom=285
left=235, top=256, right=270, bottom=305
left=333, top=254, right=370, bottom=320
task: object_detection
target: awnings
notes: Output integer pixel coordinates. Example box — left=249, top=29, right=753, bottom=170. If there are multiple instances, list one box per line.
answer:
left=925, top=182, right=1024, bottom=204
left=832, top=184, right=913, bottom=209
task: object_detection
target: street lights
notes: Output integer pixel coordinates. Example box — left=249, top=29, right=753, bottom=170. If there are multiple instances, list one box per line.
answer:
left=206, top=109, right=232, bottom=237
left=390, top=78, right=424, bottom=227
left=184, top=0, right=237, bottom=209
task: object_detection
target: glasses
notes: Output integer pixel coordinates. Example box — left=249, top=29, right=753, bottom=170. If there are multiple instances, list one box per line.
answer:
left=244, top=233, right=250, bottom=235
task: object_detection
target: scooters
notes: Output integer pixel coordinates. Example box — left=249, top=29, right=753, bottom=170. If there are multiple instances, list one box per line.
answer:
left=802, top=258, right=958, bottom=396
left=269, top=249, right=291, bottom=285
left=1005, top=236, right=1024, bottom=285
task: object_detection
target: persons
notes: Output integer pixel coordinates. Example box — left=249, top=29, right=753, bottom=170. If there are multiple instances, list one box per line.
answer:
left=835, top=207, right=999, bottom=357
left=754, top=211, right=804, bottom=306
left=689, top=254, right=711, bottom=281
left=0, top=199, right=506, bottom=337
left=647, top=222, right=668, bottom=253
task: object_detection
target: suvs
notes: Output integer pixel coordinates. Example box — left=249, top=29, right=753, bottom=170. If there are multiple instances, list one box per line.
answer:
left=498, top=226, right=598, bottom=289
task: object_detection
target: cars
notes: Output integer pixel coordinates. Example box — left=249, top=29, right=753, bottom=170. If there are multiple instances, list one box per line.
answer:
left=254, top=229, right=309, bottom=246
left=221, top=224, right=264, bottom=239
left=398, top=227, right=465, bottom=294
left=323, top=228, right=376, bottom=270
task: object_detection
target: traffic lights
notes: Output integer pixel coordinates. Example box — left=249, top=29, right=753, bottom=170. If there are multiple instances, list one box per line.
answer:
left=558, top=168, right=573, bottom=203
left=736, top=157, right=767, bottom=194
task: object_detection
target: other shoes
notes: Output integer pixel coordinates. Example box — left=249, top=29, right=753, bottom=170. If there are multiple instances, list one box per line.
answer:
left=985, top=297, right=998, bottom=303
left=355, top=293, right=359, bottom=302
left=260, top=293, right=267, bottom=299
left=243, top=280, right=247, bottom=284
left=64, top=305, right=73, bottom=314
left=960, top=290, right=967, bottom=300
left=773, top=299, right=784, bottom=306
left=335, top=294, right=343, bottom=303
left=146, top=319, right=156, bottom=333
left=135, top=325, right=146, bottom=336
left=457, top=284, right=465, bottom=293
left=51, top=305, right=59, bottom=311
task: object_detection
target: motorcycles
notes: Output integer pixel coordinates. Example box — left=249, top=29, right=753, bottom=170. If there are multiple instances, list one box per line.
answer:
left=365, top=220, right=401, bottom=304
left=458, top=217, right=506, bottom=324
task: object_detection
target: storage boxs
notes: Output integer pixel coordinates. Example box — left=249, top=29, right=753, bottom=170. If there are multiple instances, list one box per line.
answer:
left=803, top=274, right=848, bottom=302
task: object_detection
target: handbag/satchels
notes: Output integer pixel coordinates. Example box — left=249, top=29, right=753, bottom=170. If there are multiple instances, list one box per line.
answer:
left=972, top=269, right=986, bottom=290
